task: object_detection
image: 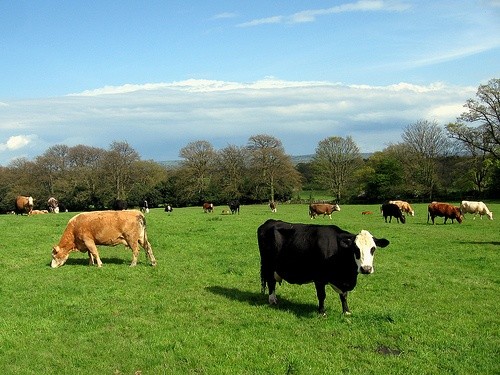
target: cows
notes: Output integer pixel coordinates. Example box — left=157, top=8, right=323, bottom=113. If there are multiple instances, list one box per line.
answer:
left=257, top=218, right=390, bottom=316
left=460, top=200, right=493, bottom=221
left=270, top=201, right=277, bottom=212
left=139, top=199, right=150, bottom=213
left=165, top=204, right=174, bottom=212
left=427, top=201, right=462, bottom=224
left=226, top=199, right=240, bottom=215
left=51, top=208, right=157, bottom=268
left=308, top=202, right=341, bottom=220
left=203, top=202, right=214, bottom=213
left=380, top=200, right=415, bottom=224
left=15, top=195, right=59, bottom=216
left=112, top=200, right=129, bottom=210
